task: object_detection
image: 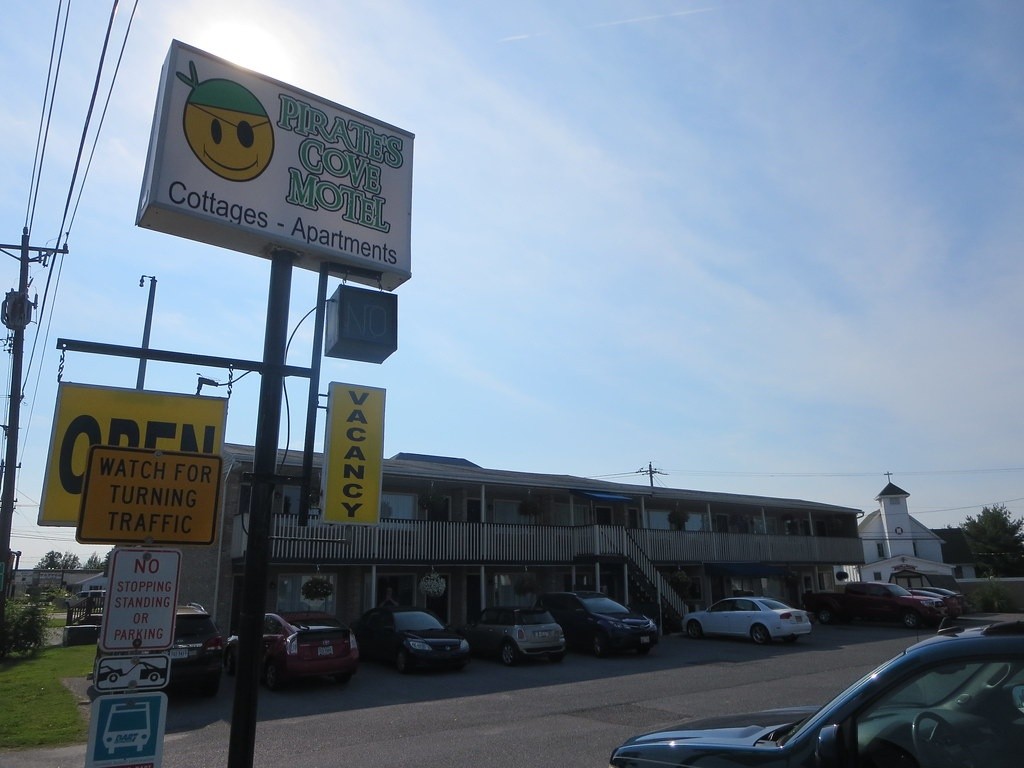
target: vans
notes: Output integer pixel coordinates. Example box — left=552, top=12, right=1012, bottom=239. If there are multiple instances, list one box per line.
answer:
left=65, top=590, right=106, bottom=609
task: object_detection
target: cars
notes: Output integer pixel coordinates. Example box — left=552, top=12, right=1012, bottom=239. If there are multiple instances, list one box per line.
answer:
left=458, top=606, right=565, bottom=665
left=681, top=596, right=811, bottom=644
left=350, top=606, right=471, bottom=675
left=224, top=611, right=359, bottom=689
left=906, top=587, right=967, bottom=616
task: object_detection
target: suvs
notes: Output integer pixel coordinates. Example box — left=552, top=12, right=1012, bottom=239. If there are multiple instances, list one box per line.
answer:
left=93, top=602, right=223, bottom=697
left=608, top=621, right=1024, bottom=768
left=534, top=591, right=658, bottom=657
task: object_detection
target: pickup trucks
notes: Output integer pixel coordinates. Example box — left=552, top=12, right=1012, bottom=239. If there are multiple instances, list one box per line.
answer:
left=804, top=582, right=944, bottom=628
